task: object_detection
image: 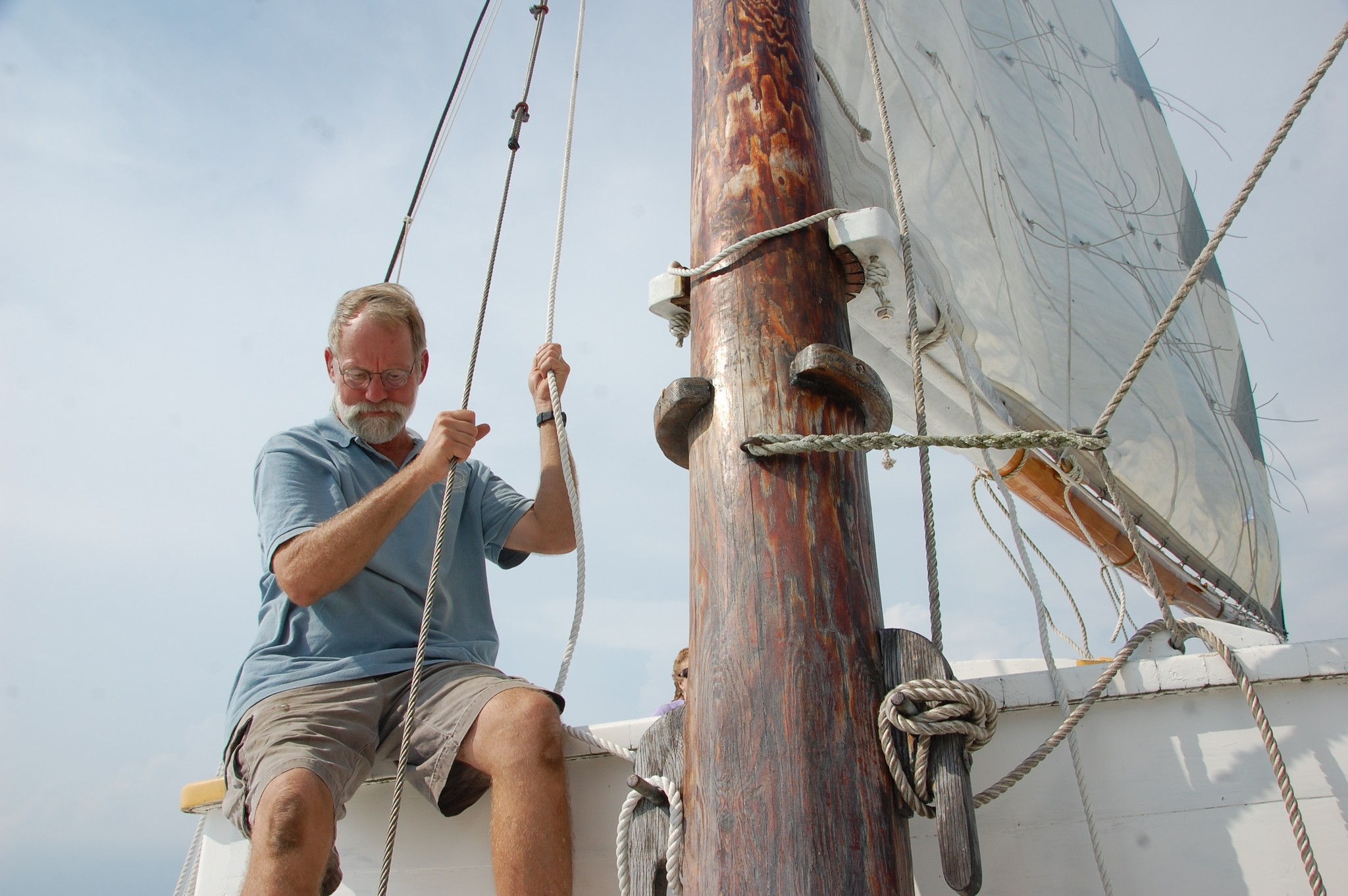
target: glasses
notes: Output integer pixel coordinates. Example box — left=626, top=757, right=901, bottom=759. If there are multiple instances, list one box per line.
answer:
left=678, top=668, right=688, bottom=679
left=333, top=352, right=417, bottom=390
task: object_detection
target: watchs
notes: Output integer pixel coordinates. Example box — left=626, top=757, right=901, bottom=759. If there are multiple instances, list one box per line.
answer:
left=535, top=412, right=567, bottom=426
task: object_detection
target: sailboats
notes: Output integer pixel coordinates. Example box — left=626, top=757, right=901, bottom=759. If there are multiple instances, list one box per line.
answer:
left=183, top=2, right=1347, bottom=895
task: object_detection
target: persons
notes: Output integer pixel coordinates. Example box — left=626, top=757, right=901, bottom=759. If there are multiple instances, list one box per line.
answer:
left=220, top=284, right=579, bottom=896
